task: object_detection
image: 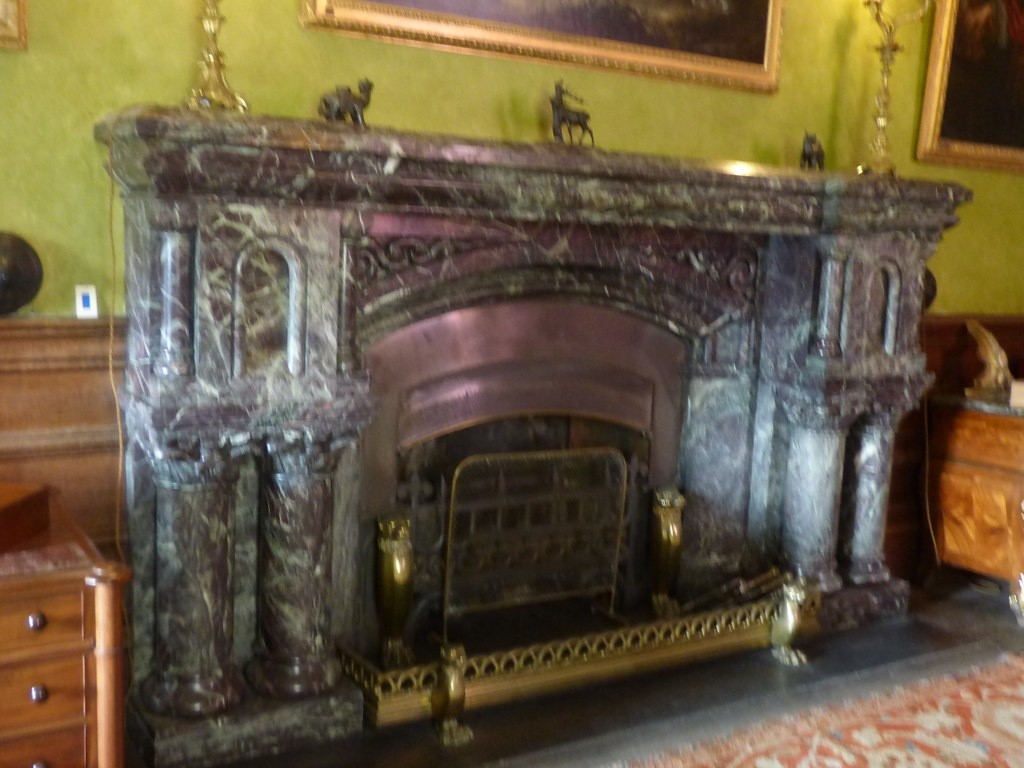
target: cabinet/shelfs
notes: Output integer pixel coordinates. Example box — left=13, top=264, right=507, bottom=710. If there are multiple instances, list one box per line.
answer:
left=0, top=522, right=129, bottom=768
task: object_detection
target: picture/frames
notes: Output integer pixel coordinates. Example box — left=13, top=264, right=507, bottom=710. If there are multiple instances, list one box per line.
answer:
left=0, top=0, right=29, bottom=50
left=916, top=0, right=1024, bottom=170
left=299, top=0, right=785, bottom=94
